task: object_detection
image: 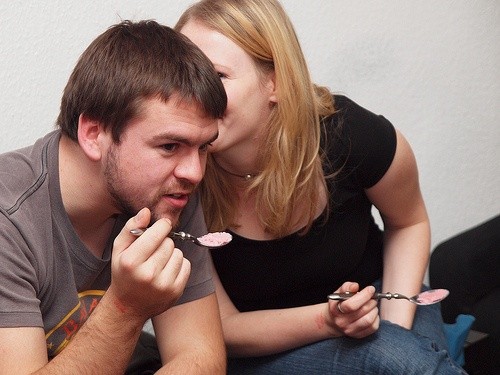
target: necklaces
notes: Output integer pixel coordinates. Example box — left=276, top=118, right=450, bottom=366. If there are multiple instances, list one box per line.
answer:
left=214, top=159, right=256, bottom=181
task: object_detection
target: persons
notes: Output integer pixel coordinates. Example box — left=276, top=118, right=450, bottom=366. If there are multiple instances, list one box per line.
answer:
left=175, top=0, right=469, bottom=375
left=0, top=19, right=228, bottom=375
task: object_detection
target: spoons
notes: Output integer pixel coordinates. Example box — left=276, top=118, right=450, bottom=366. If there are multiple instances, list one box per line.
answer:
left=129, top=228, right=234, bottom=249
left=327, top=288, right=449, bottom=305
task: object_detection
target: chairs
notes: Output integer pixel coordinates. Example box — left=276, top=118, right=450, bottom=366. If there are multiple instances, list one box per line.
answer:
left=429, top=216, right=500, bottom=375
